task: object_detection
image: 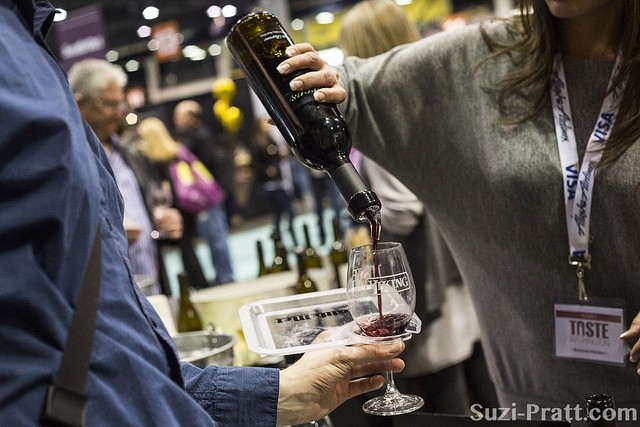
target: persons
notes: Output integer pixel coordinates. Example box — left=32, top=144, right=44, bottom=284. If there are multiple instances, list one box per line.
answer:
left=173, top=101, right=230, bottom=212
left=340, top=0, right=485, bottom=414
left=253, top=119, right=300, bottom=247
left=138, top=116, right=233, bottom=283
left=275, top=0, right=639, bottom=422
left=1, top=0, right=408, bottom=427
left=65, top=59, right=170, bottom=303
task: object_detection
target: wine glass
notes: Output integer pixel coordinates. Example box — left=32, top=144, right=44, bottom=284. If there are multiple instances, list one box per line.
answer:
left=144, top=180, right=175, bottom=241
left=345, top=240, right=424, bottom=415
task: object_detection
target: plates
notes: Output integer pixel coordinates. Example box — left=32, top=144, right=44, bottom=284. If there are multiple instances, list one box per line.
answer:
left=239, top=283, right=422, bottom=355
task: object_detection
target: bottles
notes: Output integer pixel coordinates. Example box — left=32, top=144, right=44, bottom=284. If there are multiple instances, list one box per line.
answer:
left=302, top=224, right=319, bottom=268
left=269, top=230, right=287, bottom=273
left=257, top=241, right=269, bottom=275
left=294, top=253, right=317, bottom=291
left=225, top=10, right=380, bottom=220
left=175, top=269, right=202, bottom=332
left=329, top=222, right=349, bottom=288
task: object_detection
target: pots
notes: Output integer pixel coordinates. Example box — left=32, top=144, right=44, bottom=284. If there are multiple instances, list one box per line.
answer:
left=162, top=329, right=234, bottom=369
